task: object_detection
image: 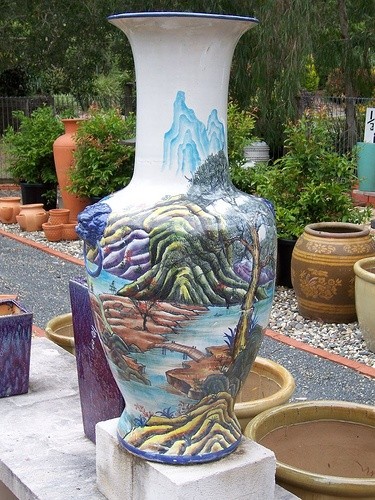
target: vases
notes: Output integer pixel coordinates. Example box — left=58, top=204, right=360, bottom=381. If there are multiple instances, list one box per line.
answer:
left=48, top=208, right=72, bottom=224
left=290, top=222, right=374, bottom=323
left=0, top=298, right=35, bottom=398
left=54, top=117, right=93, bottom=223
left=41, top=222, right=62, bottom=242
left=44, top=312, right=75, bottom=357
left=0, top=197, right=22, bottom=224
left=68, top=278, right=126, bottom=445
left=15, top=203, right=49, bottom=233
left=243, top=400, right=375, bottom=500
left=233, top=354, right=297, bottom=434
left=354, top=256, right=374, bottom=356
left=74, top=10, right=280, bottom=467
left=62, top=223, right=80, bottom=242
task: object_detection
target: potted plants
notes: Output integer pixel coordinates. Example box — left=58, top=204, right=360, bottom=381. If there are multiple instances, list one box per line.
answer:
left=73, top=110, right=136, bottom=204
left=255, top=104, right=370, bottom=289
left=0, top=105, right=65, bottom=212
